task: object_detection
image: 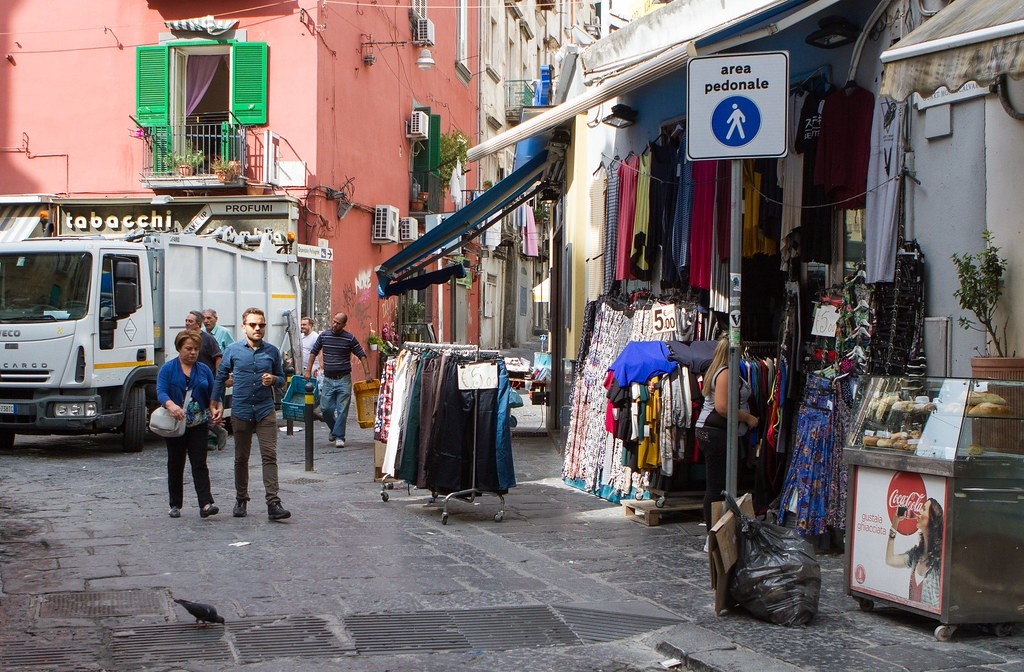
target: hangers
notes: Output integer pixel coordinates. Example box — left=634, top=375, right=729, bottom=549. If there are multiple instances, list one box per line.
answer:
left=601, top=284, right=837, bottom=415
left=404, top=339, right=503, bottom=362
left=792, top=66, right=860, bottom=96
left=593, top=119, right=687, bottom=176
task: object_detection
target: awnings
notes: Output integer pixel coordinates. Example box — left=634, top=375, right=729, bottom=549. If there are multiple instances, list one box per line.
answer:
left=376, top=150, right=559, bottom=299
left=467, top=0, right=842, bottom=163
left=877, top=0, right=1024, bottom=122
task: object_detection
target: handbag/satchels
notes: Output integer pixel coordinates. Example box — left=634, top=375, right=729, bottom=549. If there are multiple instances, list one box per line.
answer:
left=719, top=493, right=820, bottom=627
left=149, top=407, right=186, bottom=437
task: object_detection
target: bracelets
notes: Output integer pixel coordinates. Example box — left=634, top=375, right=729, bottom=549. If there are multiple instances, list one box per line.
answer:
left=888, top=528, right=896, bottom=539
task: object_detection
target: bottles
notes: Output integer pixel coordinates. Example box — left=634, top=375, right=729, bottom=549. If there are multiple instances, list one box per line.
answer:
left=897, top=507, right=920, bottom=519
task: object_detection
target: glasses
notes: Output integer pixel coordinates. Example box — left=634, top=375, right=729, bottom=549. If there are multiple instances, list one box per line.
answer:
left=245, top=321, right=267, bottom=329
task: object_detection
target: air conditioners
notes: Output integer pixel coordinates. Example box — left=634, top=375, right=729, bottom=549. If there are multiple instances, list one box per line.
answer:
left=411, top=111, right=429, bottom=136
left=373, top=205, right=398, bottom=242
left=416, top=18, right=435, bottom=45
left=400, top=217, right=419, bottom=240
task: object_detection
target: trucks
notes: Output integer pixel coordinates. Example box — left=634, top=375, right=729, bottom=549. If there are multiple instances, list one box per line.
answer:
left=0, top=228, right=301, bottom=454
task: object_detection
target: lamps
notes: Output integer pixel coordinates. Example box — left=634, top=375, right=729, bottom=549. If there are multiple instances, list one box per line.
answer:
left=331, top=191, right=344, bottom=198
left=805, top=15, right=860, bottom=49
left=363, top=40, right=434, bottom=72
left=602, top=105, right=637, bottom=130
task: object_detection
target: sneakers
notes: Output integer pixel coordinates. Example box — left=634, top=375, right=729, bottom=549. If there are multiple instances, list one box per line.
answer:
left=233, top=497, right=247, bottom=517
left=269, top=501, right=291, bottom=519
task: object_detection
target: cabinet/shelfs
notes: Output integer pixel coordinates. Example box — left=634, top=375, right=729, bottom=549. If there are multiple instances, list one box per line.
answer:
left=843, top=376, right=1024, bottom=640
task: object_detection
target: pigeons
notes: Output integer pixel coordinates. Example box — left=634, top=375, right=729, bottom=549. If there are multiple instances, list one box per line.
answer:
left=174, top=599, right=225, bottom=627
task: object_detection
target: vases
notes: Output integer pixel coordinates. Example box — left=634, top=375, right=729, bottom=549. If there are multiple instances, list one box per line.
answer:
left=246, top=181, right=266, bottom=196
left=411, top=200, right=423, bottom=211
left=419, top=192, right=429, bottom=201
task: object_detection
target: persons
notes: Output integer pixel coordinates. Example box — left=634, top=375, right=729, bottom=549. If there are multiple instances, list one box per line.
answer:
left=696, top=331, right=760, bottom=551
left=210, top=307, right=291, bottom=521
left=303, top=313, right=372, bottom=447
left=202, top=308, right=235, bottom=451
left=885, top=498, right=943, bottom=610
left=156, top=330, right=223, bottom=518
left=186, top=310, right=228, bottom=450
left=301, top=317, right=323, bottom=379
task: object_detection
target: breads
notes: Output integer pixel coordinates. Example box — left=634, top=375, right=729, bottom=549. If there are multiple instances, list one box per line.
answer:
left=863, top=397, right=937, bottom=451
left=964, top=391, right=1011, bottom=417
left=967, top=444, right=983, bottom=455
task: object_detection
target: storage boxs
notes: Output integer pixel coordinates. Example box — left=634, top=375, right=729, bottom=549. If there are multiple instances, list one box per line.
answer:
left=352, top=379, right=381, bottom=429
left=280, top=375, right=321, bottom=422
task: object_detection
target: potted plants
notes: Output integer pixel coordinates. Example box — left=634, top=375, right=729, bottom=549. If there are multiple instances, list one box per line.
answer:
left=951, top=229, right=1024, bottom=452
left=409, top=303, right=426, bottom=322
left=210, top=156, right=238, bottom=184
left=167, top=142, right=207, bottom=177
left=369, top=336, right=380, bottom=351
left=484, top=181, right=493, bottom=189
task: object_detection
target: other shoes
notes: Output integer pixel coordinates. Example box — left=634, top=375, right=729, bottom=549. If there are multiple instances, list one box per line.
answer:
left=207, top=438, right=217, bottom=449
left=199, top=504, right=218, bottom=518
left=329, top=430, right=336, bottom=442
left=218, top=430, right=228, bottom=450
left=169, top=504, right=181, bottom=518
left=335, top=436, right=345, bottom=448
